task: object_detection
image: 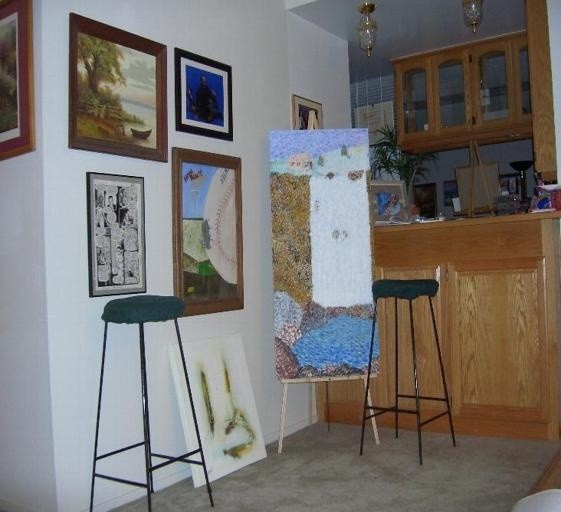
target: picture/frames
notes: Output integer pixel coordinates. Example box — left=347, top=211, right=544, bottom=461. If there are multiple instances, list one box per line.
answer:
left=290, top=93, right=323, bottom=127
left=171, top=147, right=246, bottom=318
left=175, top=44, right=235, bottom=141
left=69, top=12, right=168, bottom=164
left=0, top=0, right=37, bottom=164
left=87, top=173, right=148, bottom=296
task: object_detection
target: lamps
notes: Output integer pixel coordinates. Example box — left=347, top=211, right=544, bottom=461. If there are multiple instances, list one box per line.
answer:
left=463, top=2, right=484, bottom=36
left=354, top=4, right=380, bottom=59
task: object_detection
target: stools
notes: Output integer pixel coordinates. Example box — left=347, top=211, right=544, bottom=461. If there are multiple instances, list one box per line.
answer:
left=88, top=297, right=213, bottom=511
left=357, top=278, right=457, bottom=463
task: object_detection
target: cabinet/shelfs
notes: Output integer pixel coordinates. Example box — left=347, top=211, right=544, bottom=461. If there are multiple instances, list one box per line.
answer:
left=313, top=206, right=560, bottom=441
left=390, top=28, right=473, bottom=155
left=474, top=28, right=534, bottom=148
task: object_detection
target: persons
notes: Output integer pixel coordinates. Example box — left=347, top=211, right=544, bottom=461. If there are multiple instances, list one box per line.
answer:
left=98, top=186, right=132, bottom=226
left=196, top=74, right=217, bottom=123
